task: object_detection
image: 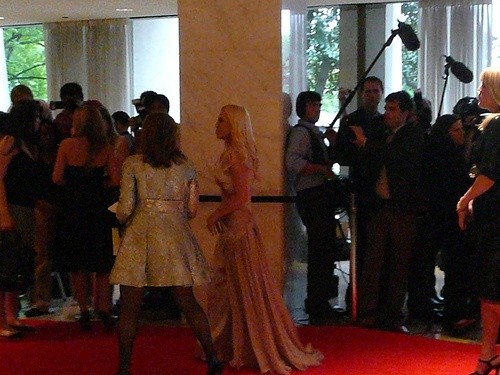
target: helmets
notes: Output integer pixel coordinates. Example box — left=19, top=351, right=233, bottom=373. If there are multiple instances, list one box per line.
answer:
left=453, top=97, right=481, bottom=115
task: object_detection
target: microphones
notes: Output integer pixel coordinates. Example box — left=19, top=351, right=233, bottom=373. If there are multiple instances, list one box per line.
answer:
left=446, top=56, right=473, bottom=83
left=399, top=22, right=420, bottom=51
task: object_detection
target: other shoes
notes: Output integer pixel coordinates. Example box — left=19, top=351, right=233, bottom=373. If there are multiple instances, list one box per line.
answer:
left=358, top=309, right=410, bottom=335
left=24, top=306, right=53, bottom=317
left=305, top=308, right=346, bottom=325
left=0, top=324, right=36, bottom=340
left=406, top=297, right=445, bottom=323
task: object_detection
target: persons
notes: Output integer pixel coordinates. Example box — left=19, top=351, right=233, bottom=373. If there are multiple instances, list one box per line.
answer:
left=284, top=76, right=500, bottom=343
left=456, top=67, right=500, bottom=375
left=108, top=111, right=224, bottom=375
left=0, top=82, right=182, bottom=339
left=195, top=104, right=324, bottom=375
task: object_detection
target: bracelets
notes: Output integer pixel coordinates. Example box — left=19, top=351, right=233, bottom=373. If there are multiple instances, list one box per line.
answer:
left=457, top=204, right=469, bottom=213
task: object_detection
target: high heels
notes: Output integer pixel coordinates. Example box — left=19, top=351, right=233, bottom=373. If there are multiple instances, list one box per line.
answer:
left=77, top=310, right=92, bottom=331
left=203, top=359, right=223, bottom=375
left=99, top=310, right=115, bottom=330
left=466, top=354, right=500, bottom=375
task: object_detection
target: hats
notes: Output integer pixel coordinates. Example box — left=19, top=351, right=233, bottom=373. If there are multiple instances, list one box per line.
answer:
left=132, top=91, right=158, bottom=106
